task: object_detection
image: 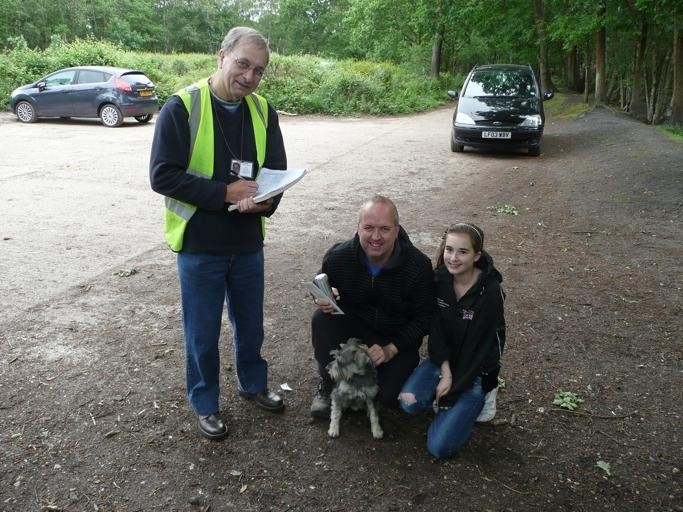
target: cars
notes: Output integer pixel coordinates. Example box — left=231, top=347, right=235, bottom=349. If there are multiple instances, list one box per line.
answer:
left=9, top=66, right=158, bottom=128
left=447, top=62, right=553, bottom=156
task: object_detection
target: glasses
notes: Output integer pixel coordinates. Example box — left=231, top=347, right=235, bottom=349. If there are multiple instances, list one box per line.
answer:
left=231, top=56, right=263, bottom=79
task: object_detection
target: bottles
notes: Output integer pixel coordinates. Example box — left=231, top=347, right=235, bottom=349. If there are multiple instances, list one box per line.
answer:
left=314, top=272, right=338, bottom=305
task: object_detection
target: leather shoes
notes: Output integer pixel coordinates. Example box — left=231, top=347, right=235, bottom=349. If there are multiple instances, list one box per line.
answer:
left=309, top=391, right=332, bottom=419
left=239, top=389, right=284, bottom=411
left=197, top=414, right=229, bottom=440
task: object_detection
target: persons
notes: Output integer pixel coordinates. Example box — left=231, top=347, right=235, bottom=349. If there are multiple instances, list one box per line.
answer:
left=311, top=196, right=434, bottom=418
left=397, top=222, right=506, bottom=458
left=149, top=26, right=287, bottom=440
left=233, top=163, right=240, bottom=175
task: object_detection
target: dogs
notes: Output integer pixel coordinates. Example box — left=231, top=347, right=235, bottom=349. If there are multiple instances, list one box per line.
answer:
left=326, top=338, right=383, bottom=439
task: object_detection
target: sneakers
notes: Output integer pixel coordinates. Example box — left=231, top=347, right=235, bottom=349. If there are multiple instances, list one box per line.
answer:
left=477, top=385, right=498, bottom=423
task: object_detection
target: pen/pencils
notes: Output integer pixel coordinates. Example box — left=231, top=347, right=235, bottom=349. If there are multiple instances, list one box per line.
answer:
left=230, top=170, right=246, bottom=181
